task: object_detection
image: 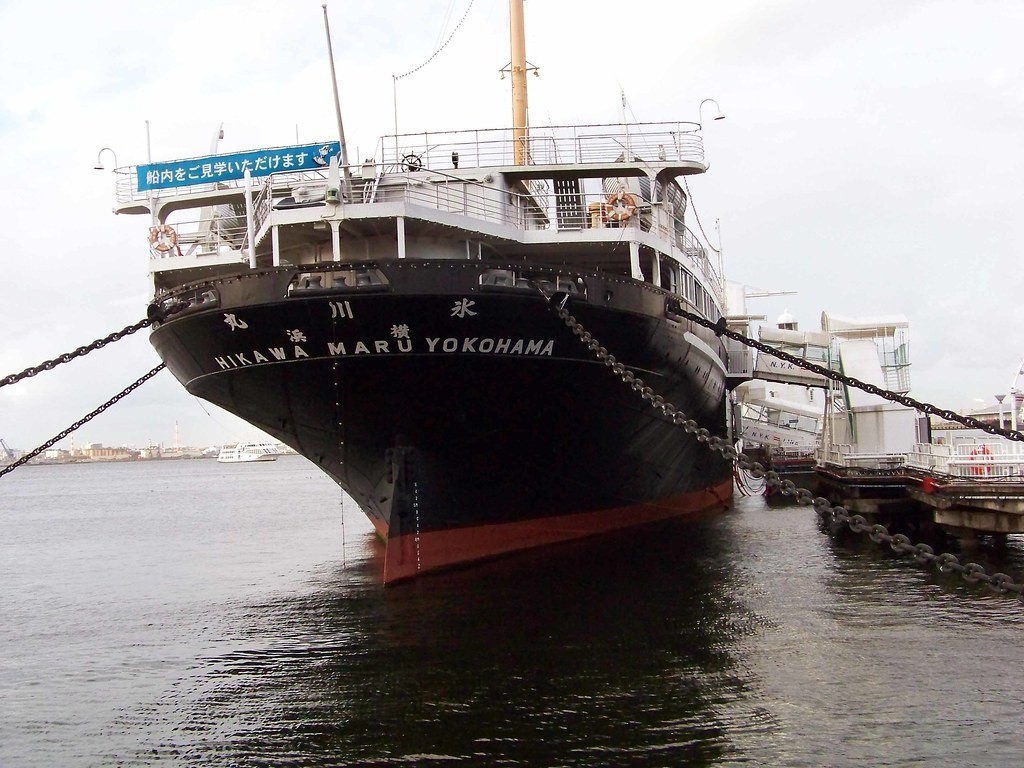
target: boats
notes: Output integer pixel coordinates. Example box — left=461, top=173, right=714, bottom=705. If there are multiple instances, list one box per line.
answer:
left=90, top=1, right=1022, bottom=588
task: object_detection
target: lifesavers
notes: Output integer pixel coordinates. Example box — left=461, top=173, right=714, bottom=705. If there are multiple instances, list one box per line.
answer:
left=968, top=446, right=994, bottom=473
left=606, top=192, right=634, bottom=221
left=150, top=225, right=177, bottom=251
left=776, top=447, right=784, bottom=454
